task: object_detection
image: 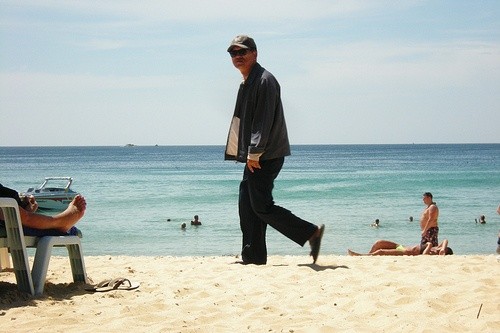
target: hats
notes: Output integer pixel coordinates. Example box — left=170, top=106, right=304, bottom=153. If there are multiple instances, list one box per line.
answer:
left=227, top=35, right=256, bottom=52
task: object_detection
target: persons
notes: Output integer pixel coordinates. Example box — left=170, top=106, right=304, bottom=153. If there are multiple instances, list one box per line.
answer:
left=0, top=183, right=89, bottom=234
left=347, top=239, right=454, bottom=256
left=497, top=230, right=500, bottom=245
left=166, top=214, right=202, bottom=229
left=224, top=34, right=325, bottom=265
left=475, top=215, right=487, bottom=225
left=497, top=205, right=500, bottom=215
left=419, top=192, right=440, bottom=247
left=408, top=216, right=414, bottom=222
left=370, top=219, right=381, bottom=228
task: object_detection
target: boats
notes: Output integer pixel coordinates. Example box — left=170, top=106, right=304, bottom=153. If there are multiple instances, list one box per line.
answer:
left=19, top=177, right=79, bottom=213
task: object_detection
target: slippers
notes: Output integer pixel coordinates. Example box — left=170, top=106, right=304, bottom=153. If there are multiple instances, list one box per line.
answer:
left=310, top=224, right=325, bottom=264
left=96, top=277, right=139, bottom=292
left=84, top=280, right=110, bottom=292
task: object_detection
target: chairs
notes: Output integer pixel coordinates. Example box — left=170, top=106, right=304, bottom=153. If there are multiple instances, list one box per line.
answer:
left=0, top=198, right=89, bottom=297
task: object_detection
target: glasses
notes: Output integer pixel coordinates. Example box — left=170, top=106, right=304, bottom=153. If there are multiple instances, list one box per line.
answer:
left=229, top=49, right=248, bottom=57
